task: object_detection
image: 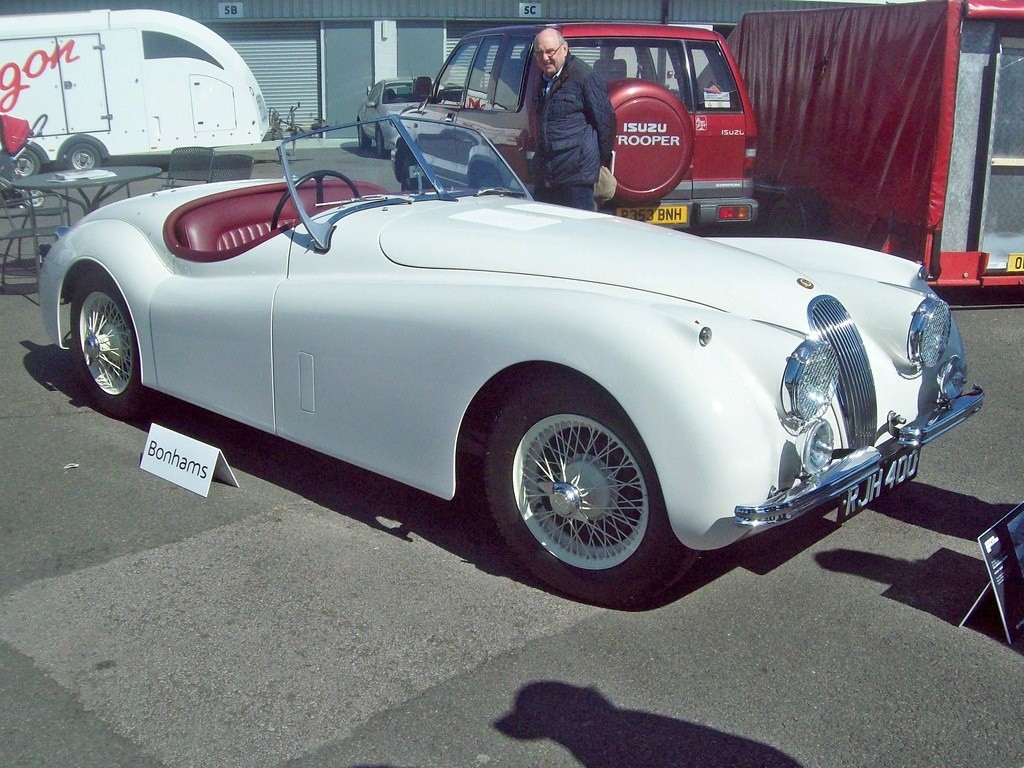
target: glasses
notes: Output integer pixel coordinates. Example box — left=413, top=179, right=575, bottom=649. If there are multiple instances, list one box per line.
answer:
left=531, top=43, right=565, bottom=58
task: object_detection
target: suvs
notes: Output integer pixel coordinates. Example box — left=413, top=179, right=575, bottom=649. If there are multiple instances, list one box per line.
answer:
left=400, top=21, right=758, bottom=238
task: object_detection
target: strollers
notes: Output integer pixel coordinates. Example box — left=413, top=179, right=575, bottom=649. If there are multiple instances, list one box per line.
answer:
left=0, top=114, right=49, bottom=209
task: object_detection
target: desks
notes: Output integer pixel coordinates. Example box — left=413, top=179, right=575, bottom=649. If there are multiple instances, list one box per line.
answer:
left=11, top=166, right=162, bottom=218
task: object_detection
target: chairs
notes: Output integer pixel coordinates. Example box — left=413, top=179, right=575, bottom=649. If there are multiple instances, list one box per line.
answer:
left=385, top=89, right=396, bottom=100
left=593, top=59, right=627, bottom=83
left=151, top=146, right=214, bottom=191
left=0, top=190, right=67, bottom=287
left=494, top=58, right=522, bottom=105
left=0, top=149, right=70, bottom=267
left=210, top=155, right=255, bottom=184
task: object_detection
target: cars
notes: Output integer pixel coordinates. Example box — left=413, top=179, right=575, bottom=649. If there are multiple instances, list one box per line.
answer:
left=357, top=78, right=459, bottom=160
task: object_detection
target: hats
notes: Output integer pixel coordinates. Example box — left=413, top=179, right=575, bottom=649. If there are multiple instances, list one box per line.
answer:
left=594, top=166, right=617, bottom=212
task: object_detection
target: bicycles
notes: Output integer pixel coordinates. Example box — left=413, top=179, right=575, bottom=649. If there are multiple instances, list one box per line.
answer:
left=268, top=101, right=327, bottom=142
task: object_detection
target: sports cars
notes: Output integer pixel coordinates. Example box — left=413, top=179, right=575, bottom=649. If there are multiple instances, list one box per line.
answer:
left=38, top=114, right=984, bottom=612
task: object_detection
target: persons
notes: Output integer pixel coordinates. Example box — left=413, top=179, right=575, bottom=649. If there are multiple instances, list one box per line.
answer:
left=535, top=29, right=617, bottom=212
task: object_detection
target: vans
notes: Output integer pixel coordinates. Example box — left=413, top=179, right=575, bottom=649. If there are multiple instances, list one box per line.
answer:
left=0, top=8, right=271, bottom=197
left=675, top=0, right=1024, bottom=305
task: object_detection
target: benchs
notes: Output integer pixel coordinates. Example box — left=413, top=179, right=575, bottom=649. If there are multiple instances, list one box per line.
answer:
left=178, top=187, right=389, bottom=251
